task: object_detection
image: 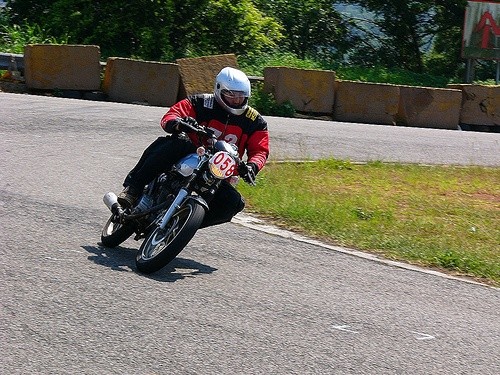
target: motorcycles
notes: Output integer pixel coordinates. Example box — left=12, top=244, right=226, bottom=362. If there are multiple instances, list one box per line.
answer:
left=101, top=116, right=256, bottom=275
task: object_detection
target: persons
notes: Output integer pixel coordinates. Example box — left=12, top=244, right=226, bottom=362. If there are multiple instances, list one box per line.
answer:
left=117, top=66, right=270, bottom=230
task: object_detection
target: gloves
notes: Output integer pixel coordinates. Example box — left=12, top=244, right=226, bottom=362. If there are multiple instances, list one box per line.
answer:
left=178, top=116, right=198, bottom=132
left=243, top=163, right=258, bottom=185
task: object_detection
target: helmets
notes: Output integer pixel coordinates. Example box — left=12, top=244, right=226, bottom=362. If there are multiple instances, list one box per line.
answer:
left=214, top=67, right=251, bottom=115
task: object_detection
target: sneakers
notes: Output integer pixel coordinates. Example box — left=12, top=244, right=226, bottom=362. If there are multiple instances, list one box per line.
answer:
left=118, top=186, right=144, bottom=208
